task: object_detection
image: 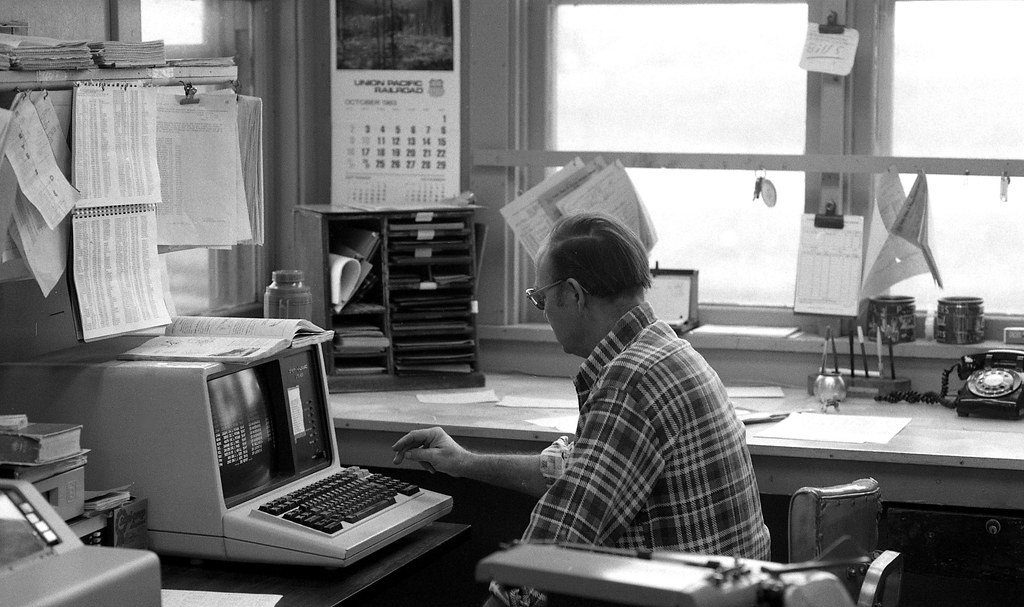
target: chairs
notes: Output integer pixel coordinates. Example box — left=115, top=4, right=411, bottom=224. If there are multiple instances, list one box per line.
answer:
left=788, top=479, right=885, bottom=570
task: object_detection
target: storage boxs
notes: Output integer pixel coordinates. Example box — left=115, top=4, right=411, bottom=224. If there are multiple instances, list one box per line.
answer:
left=643, top=262, right=700, bottom=336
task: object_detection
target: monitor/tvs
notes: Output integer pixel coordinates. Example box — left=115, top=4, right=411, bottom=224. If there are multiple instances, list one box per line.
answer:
left=206, top=366, right=279, bottom=498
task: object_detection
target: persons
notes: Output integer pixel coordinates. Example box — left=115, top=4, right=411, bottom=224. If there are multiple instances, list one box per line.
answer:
left=392, top=213, right=771, bottom=607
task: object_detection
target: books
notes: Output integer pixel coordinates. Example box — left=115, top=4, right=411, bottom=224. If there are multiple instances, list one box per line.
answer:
left=0, top=213, right=474, bottom=519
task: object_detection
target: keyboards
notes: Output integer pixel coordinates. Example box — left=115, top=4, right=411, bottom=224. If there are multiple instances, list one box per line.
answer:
left=258, top=466, right=420, bottom=534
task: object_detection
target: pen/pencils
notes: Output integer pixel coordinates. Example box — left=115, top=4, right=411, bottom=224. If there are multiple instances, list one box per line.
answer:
left=815, top=325, right=897, bottom=377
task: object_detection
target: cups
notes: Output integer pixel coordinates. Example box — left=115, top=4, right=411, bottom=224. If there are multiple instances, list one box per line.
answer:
left=937, top=297, right=985, bottom=344
left=867, top=295, right=916, bottom=345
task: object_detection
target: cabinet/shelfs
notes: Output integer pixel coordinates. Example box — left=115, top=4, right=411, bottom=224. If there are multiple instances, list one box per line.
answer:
left=290, top=203, right=486, bottom=393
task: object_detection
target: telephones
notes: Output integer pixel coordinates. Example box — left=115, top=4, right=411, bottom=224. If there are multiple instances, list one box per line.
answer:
left=949, top=348, right=1024, bottom=420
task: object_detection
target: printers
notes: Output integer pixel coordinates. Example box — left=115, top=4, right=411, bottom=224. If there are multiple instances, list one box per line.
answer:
left=0, top=479, right=161, bottom=607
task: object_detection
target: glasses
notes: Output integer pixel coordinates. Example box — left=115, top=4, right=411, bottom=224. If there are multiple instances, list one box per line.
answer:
left=525, top=278, right=591, bottom=310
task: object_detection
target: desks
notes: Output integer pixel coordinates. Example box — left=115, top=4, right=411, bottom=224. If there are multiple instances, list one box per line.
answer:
left=93, top=522, right=483, bottom=607
left=331, top=374, right=1024, bottom=510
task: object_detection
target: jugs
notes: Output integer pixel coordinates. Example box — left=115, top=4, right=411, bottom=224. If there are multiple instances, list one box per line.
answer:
left=264, top=270, right=312, bottom=321
